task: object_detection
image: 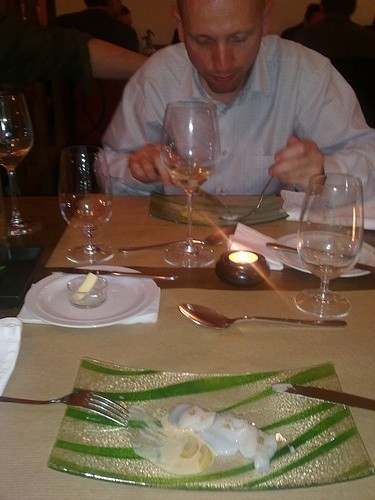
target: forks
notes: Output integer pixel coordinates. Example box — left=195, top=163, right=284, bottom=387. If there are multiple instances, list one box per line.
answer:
left=0, top=390, right=132, bottom=427
left=219, top=176, right=273, bottom=222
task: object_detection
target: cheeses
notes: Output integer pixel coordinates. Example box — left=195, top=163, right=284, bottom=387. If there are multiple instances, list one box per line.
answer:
left=73, top=272, right=98, bottom=300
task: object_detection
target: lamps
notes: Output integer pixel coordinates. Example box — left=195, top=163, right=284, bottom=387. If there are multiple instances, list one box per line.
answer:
left=216, top=250, right=271, bottom=287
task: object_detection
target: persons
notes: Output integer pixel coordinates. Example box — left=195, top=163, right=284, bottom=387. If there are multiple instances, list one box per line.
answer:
left=94, top=0, right=375, bottom=203
left=0, top=11, right=149, bottom=83
left=278, top=0, right=375, bottom=57
left=54, top=0, right=139, bottom=53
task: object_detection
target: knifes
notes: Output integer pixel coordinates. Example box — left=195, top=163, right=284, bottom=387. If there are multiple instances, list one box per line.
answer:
left=273, top=385, right=375, bottom=411
left=60, top=267, right=178, bottom=281
left=266, top=243, right=375, bottom=273
left=179, top=179, right=226, bottom=209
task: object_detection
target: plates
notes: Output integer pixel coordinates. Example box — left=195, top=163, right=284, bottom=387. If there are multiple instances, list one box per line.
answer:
left=273, top=231, right=375, bottom=278
left=24, top=264, right=158, bottom=328
left=148, top=191, right=289, bottom=227
left=48, top=355, right=375, bottom=492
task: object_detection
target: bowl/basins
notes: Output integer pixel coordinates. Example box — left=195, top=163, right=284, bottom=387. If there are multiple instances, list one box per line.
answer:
left=66, top=276, right=108, bottom=310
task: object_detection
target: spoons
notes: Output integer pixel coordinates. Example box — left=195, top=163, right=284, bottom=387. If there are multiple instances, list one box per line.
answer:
left=178, top=304, right=347, bottom=330
left=119, top=227, right=235, bottom=254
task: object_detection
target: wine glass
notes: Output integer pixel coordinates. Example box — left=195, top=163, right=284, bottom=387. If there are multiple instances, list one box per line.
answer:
left=160, top=100, right=221, bottom=268
left=58, top=145, right=114, bottom=264
left=293, top=173, right=364, bottom=317
left=0, top=90, right=42, bottom=239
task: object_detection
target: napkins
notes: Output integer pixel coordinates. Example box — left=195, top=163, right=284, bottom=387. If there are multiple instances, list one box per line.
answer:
left=0, top=317, right=22, bottom=396
left=279, top=190, right=375, bottom=230
left=228, top=223, right=285, bottom=271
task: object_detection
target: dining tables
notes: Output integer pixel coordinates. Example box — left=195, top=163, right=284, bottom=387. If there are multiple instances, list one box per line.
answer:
left=0, top=192, right=375, bottom=500
